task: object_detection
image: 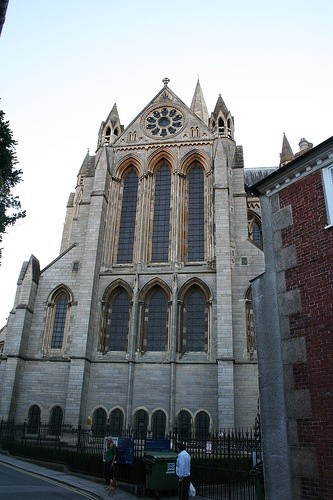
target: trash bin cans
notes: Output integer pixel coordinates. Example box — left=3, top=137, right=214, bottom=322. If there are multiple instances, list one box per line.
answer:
left=250, top=462, right=264, bottom=500
left=144, top=450, right=180, bottom=498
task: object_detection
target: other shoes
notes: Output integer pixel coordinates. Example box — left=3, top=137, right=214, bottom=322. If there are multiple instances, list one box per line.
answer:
left=104, top=484, right=109, bottom=490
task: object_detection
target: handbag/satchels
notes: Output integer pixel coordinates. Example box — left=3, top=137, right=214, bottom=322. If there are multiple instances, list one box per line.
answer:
left=188, top=482, right=197, bottom=497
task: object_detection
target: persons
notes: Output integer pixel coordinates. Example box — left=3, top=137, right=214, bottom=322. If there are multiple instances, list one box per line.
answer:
left=176, top=442, right=192, bottom=500
left=103, top=436, right=118, bottom=490
